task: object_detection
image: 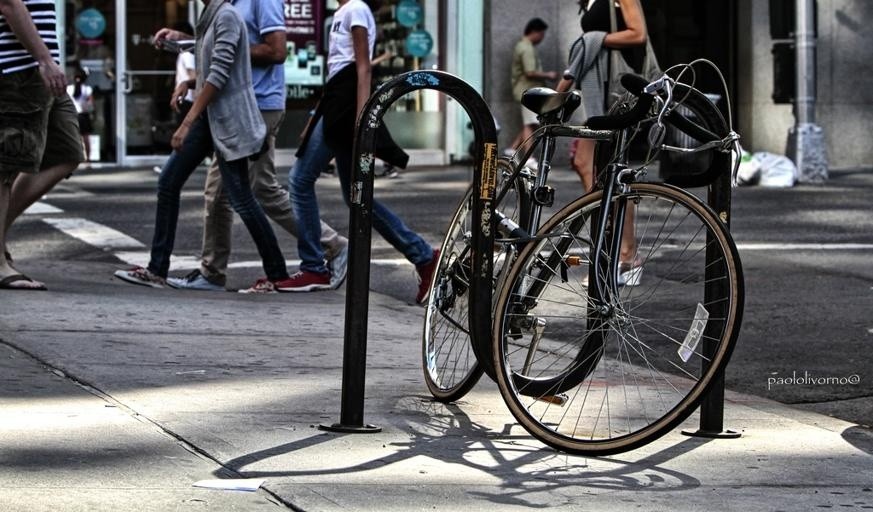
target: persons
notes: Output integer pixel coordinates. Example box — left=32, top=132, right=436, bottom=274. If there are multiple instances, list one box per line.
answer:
left=151, top=48, right=196, bottom=175
left=149, top=0, right=353, bottom=290
left=550, top=1, right=666, bottom=289
left=275, top=1, right=449, bottom=305
left=65, top=71, right=94, bottom=163
left=319, top=51, right=397, bottom=178
left=508, top=17, right=560, bottom=171
left=1, top=2, right=88, bottom=292
left=111, top=0, right=294, bottom=296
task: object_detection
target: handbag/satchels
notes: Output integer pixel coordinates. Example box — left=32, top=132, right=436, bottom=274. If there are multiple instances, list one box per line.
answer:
left=606, top=0, right=670, bottom=125
left=80, top=86, right=93, bottom=112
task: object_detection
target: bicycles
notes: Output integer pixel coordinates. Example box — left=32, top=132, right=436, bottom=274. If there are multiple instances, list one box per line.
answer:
left=414, top=55, right=752, bottom=462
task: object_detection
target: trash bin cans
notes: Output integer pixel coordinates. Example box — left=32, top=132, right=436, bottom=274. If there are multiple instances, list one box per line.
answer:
left=664, top=94, right=722, bottom=148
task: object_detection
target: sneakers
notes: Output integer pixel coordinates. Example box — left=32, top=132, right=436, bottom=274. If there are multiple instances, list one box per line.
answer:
left=165, top=269, right=225, bottom=292
left=237, top=278, right=277, bottom=294
left=414, top=249, right=441, bottom=304
left=113, top=267, right=164, bottom=289
left=327, top=240, right=348, bottom=290
left=524, top=158, right=551, bottom=173
left=274, top=269, right=330, bottom=293
left=503, top=148, right=519, bottom=166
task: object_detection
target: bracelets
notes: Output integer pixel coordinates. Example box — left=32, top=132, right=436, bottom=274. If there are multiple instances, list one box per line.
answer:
left=309, top=110, right=316, bottom=116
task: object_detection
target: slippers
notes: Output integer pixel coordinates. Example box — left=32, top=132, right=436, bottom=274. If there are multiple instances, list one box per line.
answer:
left=582, top=260, right=643, bottom=288
left=0, top=274, right=47, bottom=291
